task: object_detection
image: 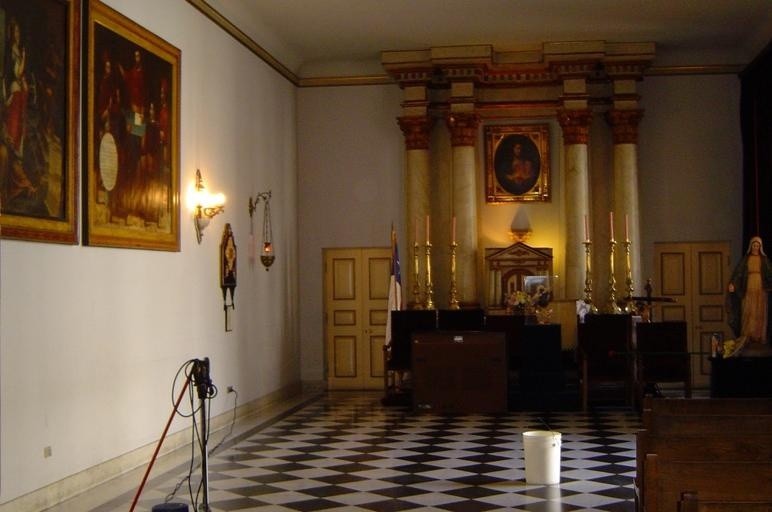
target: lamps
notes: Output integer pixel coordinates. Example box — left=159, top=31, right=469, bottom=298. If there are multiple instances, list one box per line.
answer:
left=189, top=169, right=226, bottom=244
left=247, top=183, right=275, bottom=272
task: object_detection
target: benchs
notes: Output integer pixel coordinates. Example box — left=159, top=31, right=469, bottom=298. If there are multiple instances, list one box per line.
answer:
left=633, top=396, right=772, bottom=512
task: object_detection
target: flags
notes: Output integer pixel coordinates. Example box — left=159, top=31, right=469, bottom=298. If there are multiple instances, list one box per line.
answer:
left=386, top=241, right=409, bottom=387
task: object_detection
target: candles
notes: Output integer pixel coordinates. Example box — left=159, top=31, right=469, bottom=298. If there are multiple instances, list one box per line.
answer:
left=584, top=212, right=590, bottom=242
left=424, top=215, right=430, bottom=245
left=450, top=215, right=457, bottom=246
left=624, top=214, right=629, bottom=241
left=413, top=216, right=420, bottom=244
left=609, top=212, right=614, bottom=242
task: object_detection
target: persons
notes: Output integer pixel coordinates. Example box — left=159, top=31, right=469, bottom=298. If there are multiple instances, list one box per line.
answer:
left=726, top=235, right=772, bottom=348
left=5, top=21, right=29, bottom=161
left=499, top=141, right=533, bottom=193
left=95, top=48, right=170, bottom=228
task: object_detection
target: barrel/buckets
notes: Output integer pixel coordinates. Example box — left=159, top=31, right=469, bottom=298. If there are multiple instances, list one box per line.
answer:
left=522, top=415, right=562, bottom=486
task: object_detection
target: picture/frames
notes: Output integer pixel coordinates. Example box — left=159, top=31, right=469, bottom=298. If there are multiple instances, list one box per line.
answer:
left=1, top=0, right=80, bottom=247
left=483, top=124, right=550, bottom=203
left=83, top=0, right=184, bottom=253
